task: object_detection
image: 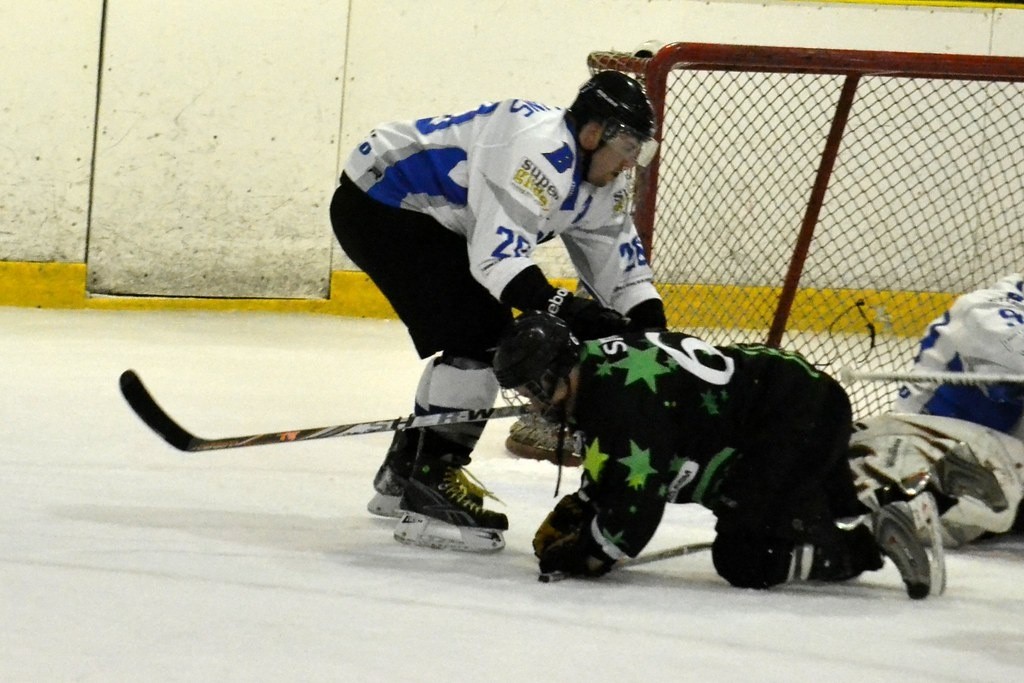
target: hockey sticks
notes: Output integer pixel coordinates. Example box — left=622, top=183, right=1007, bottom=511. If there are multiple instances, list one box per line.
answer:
left=118, top=369, right=535, bottom=455
left=840, top=365, right=1024, bottom=392
left=537, top=540, right=714, bottom=584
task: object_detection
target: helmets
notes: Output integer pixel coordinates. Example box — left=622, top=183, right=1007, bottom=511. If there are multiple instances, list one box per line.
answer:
left=568, top=71, right=660, bottom=168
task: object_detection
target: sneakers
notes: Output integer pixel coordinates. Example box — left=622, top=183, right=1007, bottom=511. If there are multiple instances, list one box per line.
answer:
left=899, top=429, right=1023, bottom=514
left=367, top=424, right=483, bottom=518
left=833, top=491, right=947, bottom=599
left=393, top=453, right=509, bottom=554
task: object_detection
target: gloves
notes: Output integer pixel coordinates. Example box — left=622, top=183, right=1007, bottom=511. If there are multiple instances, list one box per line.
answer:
left=531, top=493, right=631, bottom=579
left=545, top=288, right=627, bottom=344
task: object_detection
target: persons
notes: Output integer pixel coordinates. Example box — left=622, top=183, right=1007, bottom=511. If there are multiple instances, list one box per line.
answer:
left=493, top=313, right=1024, bottom=600
left=330, top=70, right=668, bottom=553
left=891, top=274, right=1024, bottom=444
